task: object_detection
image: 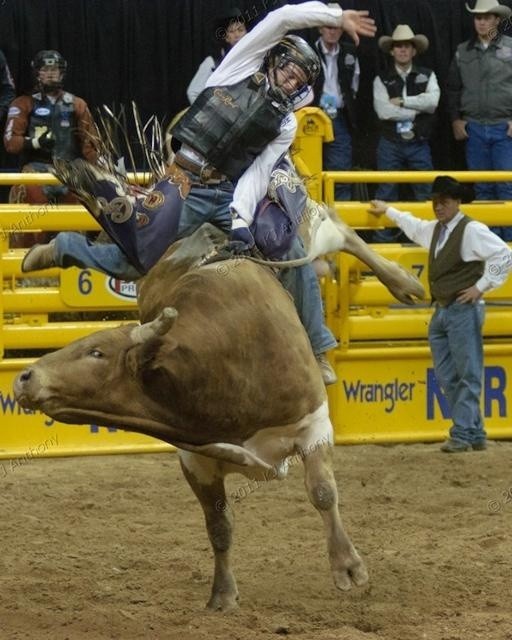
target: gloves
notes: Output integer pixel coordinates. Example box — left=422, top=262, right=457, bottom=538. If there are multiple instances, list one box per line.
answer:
left=38, top=132, right=54, bottom=149
left=229, top=213, right=257, bottom=253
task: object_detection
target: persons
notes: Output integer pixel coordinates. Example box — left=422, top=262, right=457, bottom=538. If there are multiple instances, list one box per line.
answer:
left=2, top=1, right=511, bottom=454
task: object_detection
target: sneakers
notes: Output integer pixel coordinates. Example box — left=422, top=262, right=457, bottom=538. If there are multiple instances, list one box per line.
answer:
left=441, top=439, right=489, bottom=452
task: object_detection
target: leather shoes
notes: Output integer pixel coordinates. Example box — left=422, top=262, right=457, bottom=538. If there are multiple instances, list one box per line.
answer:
left=314, top=352, right=338, bottom=385
left=22, top=239, right=59, bottom=273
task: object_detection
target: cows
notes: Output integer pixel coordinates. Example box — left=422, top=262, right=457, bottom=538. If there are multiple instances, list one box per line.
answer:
left=10, top=199, right=427, bottom=615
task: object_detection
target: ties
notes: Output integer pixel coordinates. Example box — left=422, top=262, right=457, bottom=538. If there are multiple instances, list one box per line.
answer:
left=436, top=226, right=446, bottom=255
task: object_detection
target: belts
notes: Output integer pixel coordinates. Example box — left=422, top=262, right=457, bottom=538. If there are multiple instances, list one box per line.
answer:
left=173, top=151, right=228, bottom=184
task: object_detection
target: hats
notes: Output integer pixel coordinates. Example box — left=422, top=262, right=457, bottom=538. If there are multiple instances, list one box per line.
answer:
left=377, top=22, right=429, bottom=55
left=467, top=0, right=511, bottom=26
left=427, top=176, right=462, bottom=199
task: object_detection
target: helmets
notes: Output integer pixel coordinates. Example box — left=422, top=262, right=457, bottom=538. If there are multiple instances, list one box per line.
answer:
left=264, top=34, right=321, bottom=117
left=30, top=50, right=67, bottom=94
